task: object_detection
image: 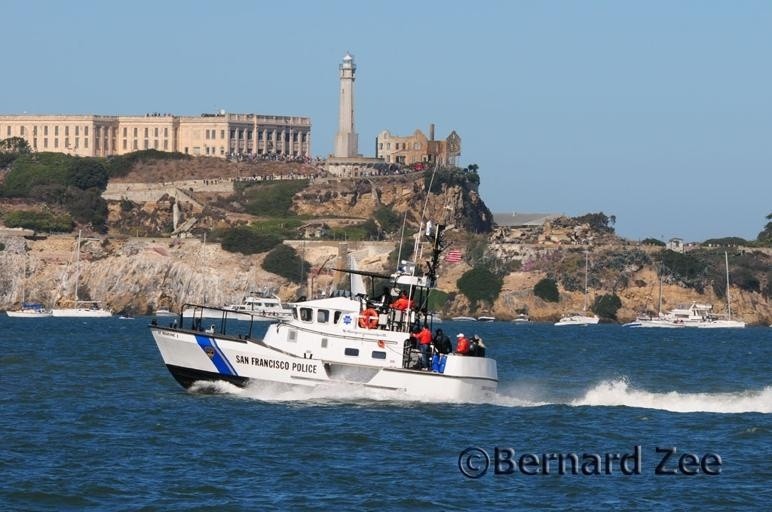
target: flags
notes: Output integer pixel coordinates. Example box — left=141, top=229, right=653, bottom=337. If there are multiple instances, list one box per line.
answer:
left=444, top=246, right=464, bottom=261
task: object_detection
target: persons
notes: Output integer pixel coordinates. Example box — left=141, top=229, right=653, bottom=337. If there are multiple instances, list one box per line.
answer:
left=365, top=286, right=486, bottom=373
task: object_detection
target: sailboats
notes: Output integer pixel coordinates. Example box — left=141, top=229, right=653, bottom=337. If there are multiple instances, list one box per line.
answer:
left=6, top=229, right=113, bottom=317
left=553, top=250, right=746, bottom=328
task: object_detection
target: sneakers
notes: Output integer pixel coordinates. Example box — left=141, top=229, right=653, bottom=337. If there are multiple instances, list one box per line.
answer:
left=422, top=367, right=428, bottom=371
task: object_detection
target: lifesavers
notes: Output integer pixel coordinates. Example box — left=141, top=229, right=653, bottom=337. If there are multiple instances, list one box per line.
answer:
left=360, top=309, right=379, bottom=328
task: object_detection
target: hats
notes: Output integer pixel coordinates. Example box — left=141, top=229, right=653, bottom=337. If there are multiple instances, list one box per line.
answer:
left=402, top=290, right=407, bottom=296
left=456, top=333, right=465, bottom=337
left=473, top=335, right=479, bottom=342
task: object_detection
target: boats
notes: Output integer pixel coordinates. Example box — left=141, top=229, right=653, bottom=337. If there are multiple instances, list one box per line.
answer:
left=511, top=313, right=532, bottom=324
left=147, top=224, right=499, bottom=398
